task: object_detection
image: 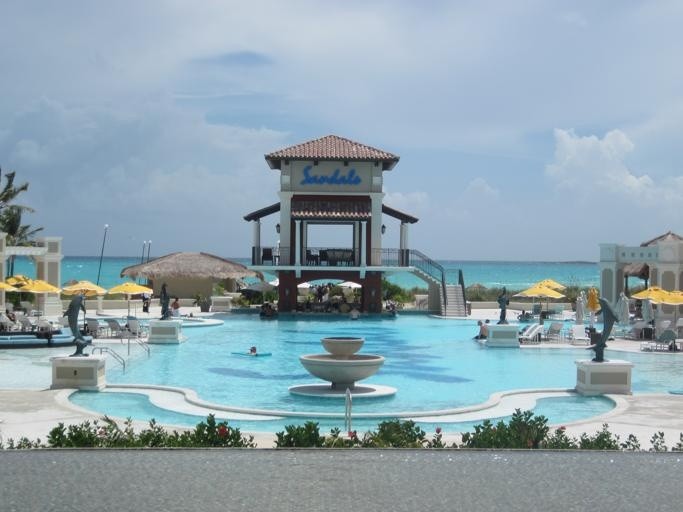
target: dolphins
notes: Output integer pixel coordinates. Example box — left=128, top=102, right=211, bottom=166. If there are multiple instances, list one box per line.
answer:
left=583, top=296, right=619, bottom=351
left=63, top=295, right=87, bottom=345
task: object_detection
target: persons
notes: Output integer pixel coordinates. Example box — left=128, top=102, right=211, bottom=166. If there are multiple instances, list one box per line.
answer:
left=517, top=310, right=527, bottom=321
left=473, top=320, right=488, bottom=340
left=171, top=297, right=181, bottom=317
left=350, top=308, right=360, bottom=320
left=309, top=282, right=335, bottom=305
left=485, top=319, right=489, bottom=323
left=190, top=313, right=193, bottom=317
left=385, top=297, right=396, bottom=316
left=259, top=303, right=274, bottom=316
left=247, top=347, right=256, bottom=354
left=141, top=292, right=150, bottom=313
left=6, top=310, right=15, bottom=322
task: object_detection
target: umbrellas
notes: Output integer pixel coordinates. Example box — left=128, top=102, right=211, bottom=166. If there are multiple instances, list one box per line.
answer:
left=336, top=280, right=362, bottom=300
left=511, top=284, right=566, bottom=316
left=630, top=285, right=683, bottom=350
left=532, top=279, right=566, bottom=317
left=106, top=282, right=153, bottom=317
left=0, top=274, right=62, bottom=332
left=587, top=287, right=601, bottom=331
left=63, top=281, right=107, bottom=334
left=269, top=279, right=313, bottom=288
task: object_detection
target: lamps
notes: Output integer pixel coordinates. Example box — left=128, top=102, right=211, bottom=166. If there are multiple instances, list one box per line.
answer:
left=276, top=223, right=280, bottom=235
left=381, top=224, right=386, bottom=234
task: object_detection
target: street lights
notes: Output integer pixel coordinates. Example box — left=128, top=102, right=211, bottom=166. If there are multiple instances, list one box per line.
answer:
left=140, top=240, right=153, bottom=265
left=97, top=224, right=109, bottom=286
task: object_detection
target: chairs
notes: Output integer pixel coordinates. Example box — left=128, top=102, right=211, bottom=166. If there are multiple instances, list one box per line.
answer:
left=519, top=323, right=683, bottom=351
left=0, top=311, right=147, bottom=338
left=262, top=249, right=273, bottom=266
left=307, top=248, right=315, bottom=266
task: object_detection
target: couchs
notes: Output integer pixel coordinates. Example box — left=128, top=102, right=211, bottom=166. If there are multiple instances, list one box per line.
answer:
left=320, top=249, right=355, bottom=267
